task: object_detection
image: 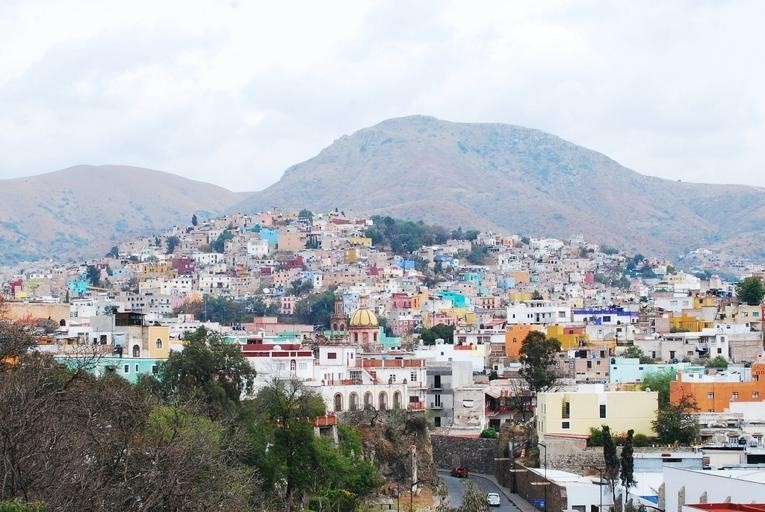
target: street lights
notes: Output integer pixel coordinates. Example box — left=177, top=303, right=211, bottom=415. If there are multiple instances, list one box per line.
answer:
left=502, top=426, right=518, bottom=494
left=530, top=441, right=547, bottom=512
left=410, top=479, right=430, bottom=512
left=581, top=463, right=605, bottom=512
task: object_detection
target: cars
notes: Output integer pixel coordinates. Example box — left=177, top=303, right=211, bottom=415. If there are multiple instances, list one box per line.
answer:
left=485, top=491, right=502, bottom=507
left=450, top=465, right=469, bottom=478
